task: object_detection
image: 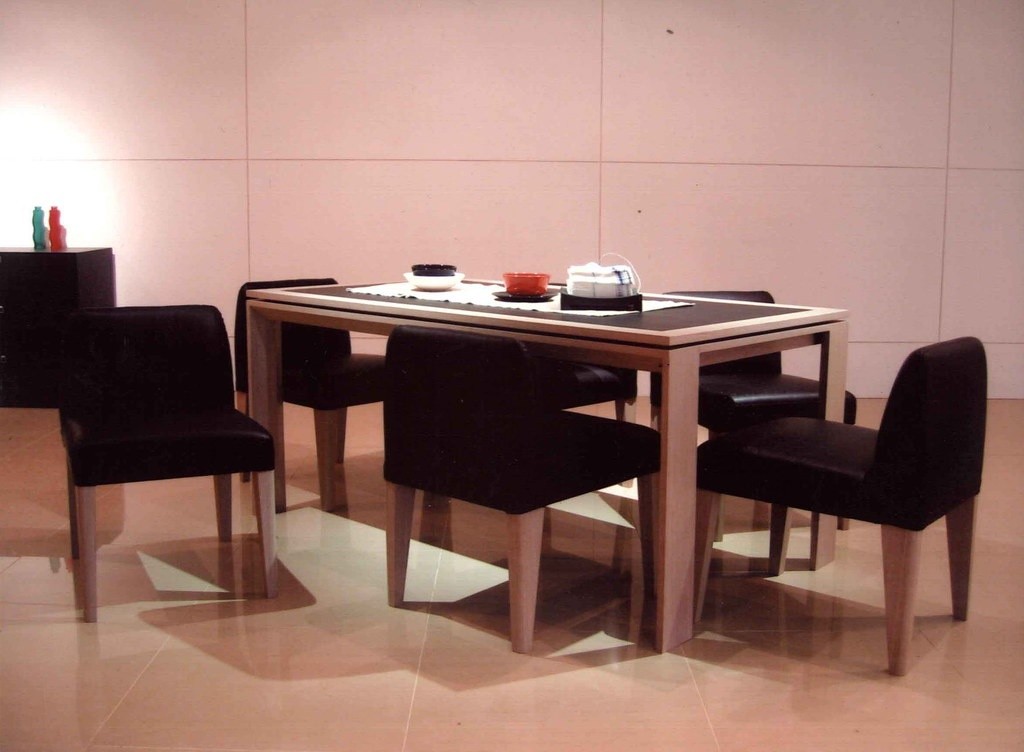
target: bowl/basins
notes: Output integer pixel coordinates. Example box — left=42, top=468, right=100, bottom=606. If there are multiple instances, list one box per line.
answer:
left=501, top=273, right=551, bottom=297
left=403, top=272, right=465, bottom=292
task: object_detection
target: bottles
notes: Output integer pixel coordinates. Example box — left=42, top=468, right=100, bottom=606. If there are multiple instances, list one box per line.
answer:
left=32, top=206, right=51, bottom=251
left=49, top=206, right=68, bottom=251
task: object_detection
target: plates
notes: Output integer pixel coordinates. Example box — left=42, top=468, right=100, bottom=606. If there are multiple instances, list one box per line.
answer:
left=491, top=291, right=558, bottom=302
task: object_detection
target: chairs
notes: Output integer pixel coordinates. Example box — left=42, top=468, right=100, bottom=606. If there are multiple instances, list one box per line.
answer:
left=382, top=325, right=660, bottom=654
left=651, top=292, right=855, bottom=542
left=234, top=279, right=387, bottom=512
left=59, top=303, right=275, bottom=621
left=690, top=335, right=989, bottom=679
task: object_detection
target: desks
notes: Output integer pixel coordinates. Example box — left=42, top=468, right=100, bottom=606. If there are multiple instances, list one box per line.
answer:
left=246, top=282, right=849, bottom=655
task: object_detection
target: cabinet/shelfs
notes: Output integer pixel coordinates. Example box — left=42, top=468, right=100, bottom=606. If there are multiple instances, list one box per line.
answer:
left=1, top=247, right=116, bottom=408
